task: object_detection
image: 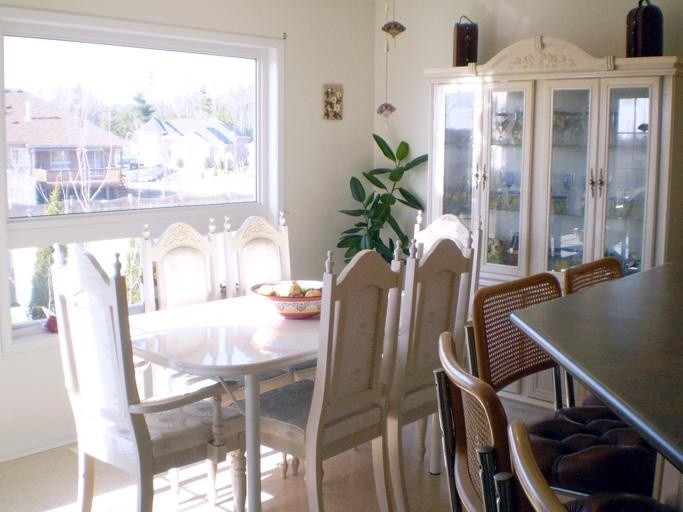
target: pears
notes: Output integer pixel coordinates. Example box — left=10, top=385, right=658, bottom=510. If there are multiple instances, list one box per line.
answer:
left=304, top=290, right=322, bottom=297
left=288, top=280, right=302, bottom=295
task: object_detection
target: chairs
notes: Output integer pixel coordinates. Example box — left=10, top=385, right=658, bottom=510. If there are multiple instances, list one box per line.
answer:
left=511, top=413, right=568, bottom=512
left=221, top=211, right=290, bottom=300
left=565, top=257, right=624, bottom=408
left=243, top=250, right=404, bottom=511
left=138, top=215, right=221, bottom=315
left=471, top=272, right=562, bottom=413
left=385, top=237, right=472, bottom=512
left=49, top=253, right=247, bottom=512
left=429, top=328, right=511, bottom=512
left=412, top=213, right=474, bottom=265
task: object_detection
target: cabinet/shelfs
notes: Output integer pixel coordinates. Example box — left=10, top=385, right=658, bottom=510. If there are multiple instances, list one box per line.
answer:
left=422, top=36, right=683, bottom=410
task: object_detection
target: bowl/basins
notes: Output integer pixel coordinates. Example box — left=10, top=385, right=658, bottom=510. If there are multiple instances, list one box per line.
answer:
left=251, top=279, right=324, bottom=320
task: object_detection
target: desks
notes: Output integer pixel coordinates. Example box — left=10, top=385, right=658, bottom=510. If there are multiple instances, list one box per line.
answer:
left=129, top=265, right=328, bottom=512
left=511, top=261, right=683, bottom=473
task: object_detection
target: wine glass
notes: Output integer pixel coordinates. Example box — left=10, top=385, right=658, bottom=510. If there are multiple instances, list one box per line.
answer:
left=499, top=172, right=516, bottom=208
left=561, top=172, right=576, bottom=212
